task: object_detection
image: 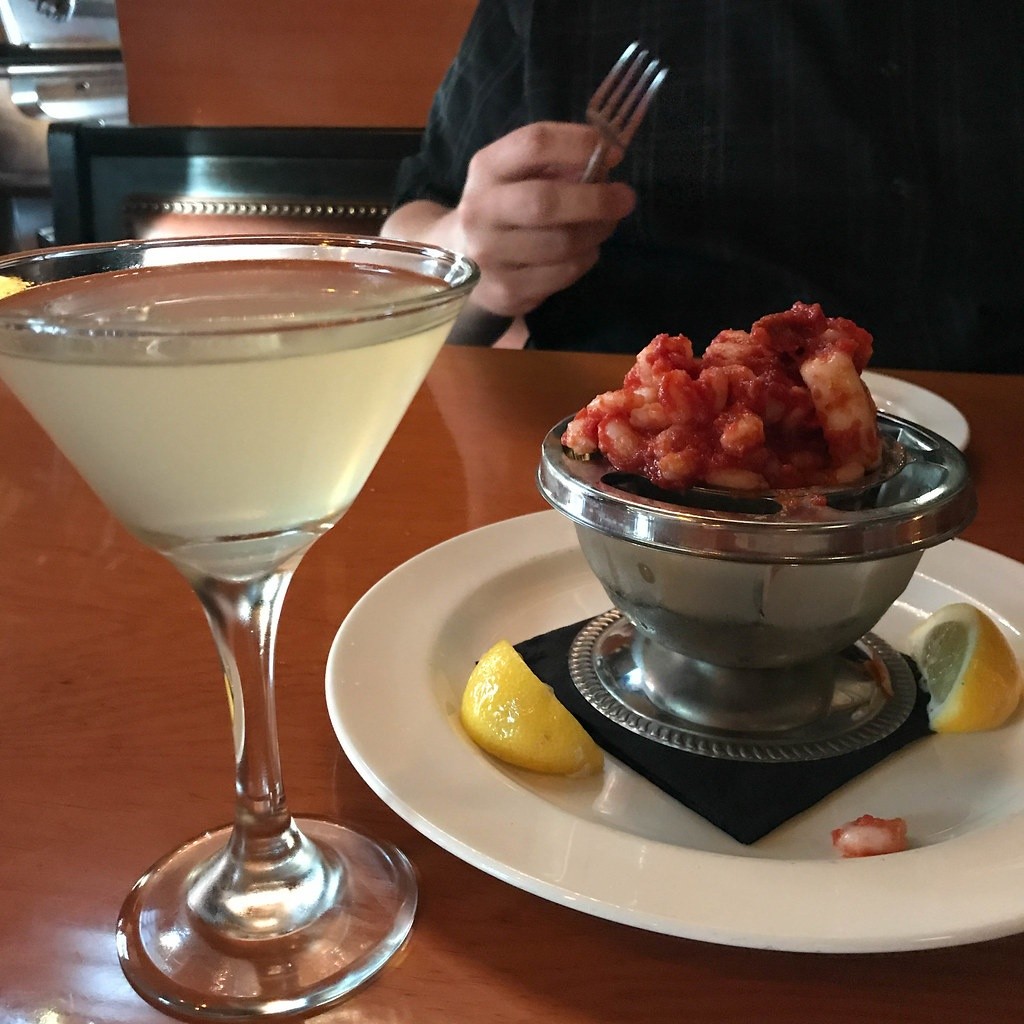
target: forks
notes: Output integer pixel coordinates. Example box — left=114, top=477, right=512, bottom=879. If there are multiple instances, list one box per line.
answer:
left=579, top=42, right=668, bottom=184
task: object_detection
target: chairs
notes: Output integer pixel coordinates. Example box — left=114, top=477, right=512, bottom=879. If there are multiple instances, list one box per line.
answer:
left=46, top=121, right=427, bottom=279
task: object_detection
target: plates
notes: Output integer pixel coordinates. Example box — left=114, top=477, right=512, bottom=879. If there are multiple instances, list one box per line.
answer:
left=325, top=510, right=1024, bottom=953
left=860, top=368, right=970, bottom=451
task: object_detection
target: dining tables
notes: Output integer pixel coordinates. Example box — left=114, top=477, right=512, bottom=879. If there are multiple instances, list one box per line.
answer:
left=0, top=342, right=1024, bottom=1024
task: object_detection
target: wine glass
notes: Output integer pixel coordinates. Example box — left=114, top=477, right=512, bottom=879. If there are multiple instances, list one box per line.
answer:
left=0, top=232, right=481, bottom=1018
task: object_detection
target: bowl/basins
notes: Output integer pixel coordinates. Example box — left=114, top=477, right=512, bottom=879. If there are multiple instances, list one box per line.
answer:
left=537, top=409, right=977, bottom=763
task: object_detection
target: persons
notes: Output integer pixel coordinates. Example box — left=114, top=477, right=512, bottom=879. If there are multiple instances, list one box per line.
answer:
left=380, top=2, right=1023, bottom=372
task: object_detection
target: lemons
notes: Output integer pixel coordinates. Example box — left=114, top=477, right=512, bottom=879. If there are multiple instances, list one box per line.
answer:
left=463, top=639, right=605, bottom=777
left=908, top=604, right=1020, bottom=731
